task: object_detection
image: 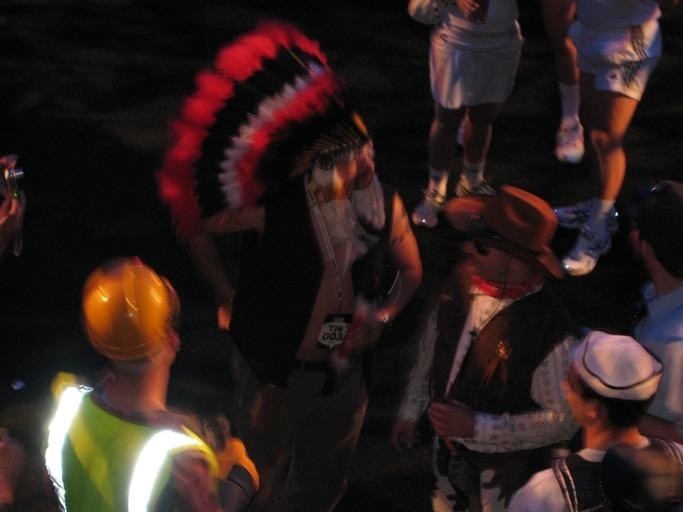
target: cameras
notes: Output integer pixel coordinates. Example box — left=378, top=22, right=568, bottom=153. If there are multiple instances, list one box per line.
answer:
left=3, top=165, right=25, bottom=201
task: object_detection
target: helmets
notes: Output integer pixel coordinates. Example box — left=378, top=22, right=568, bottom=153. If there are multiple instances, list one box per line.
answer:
left=78, top=256, right=183, bottom=362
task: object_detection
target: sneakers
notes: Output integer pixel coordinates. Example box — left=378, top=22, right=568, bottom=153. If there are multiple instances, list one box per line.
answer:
left=550, top=198, right=620, bottom=232
left=552, top=116, right=586, bottom=164
left=454, top=179, right=498, bottom=199
left=562, top=221, right=612, bottom=278
left=409, top=183, right=446, bottom=229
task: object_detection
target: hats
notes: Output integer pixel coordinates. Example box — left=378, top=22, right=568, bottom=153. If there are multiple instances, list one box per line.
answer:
left=571, top=326, right=667, bottom=404
left=444, top=182, right=568, bottom=283
left=629, top=179, right=683, bottom=265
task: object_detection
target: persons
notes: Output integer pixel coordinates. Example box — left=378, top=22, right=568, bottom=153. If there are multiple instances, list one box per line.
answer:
left=508, top=330, right=682, bottom=512
left=536, top=0, right=665, bottom=278
left=39, top=252, right=263, bottom=512
left=625, top=177, right=682, bottom=430
left=541, top=0, right=587, bottom=165
left=389, top=185, right=586, bottom=511
left=174, top=138, right=424, bottom=511
left=0, top=151, right=51, bottom=512
left=403, top=0, right=522, bottom=227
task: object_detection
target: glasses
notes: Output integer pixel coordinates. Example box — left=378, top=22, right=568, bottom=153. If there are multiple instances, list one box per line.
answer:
left=472, top=237, right=514, bottom=259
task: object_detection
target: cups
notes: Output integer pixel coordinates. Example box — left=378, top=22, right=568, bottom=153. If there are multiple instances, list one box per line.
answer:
left=202, top=417, right=232, bottom=455
